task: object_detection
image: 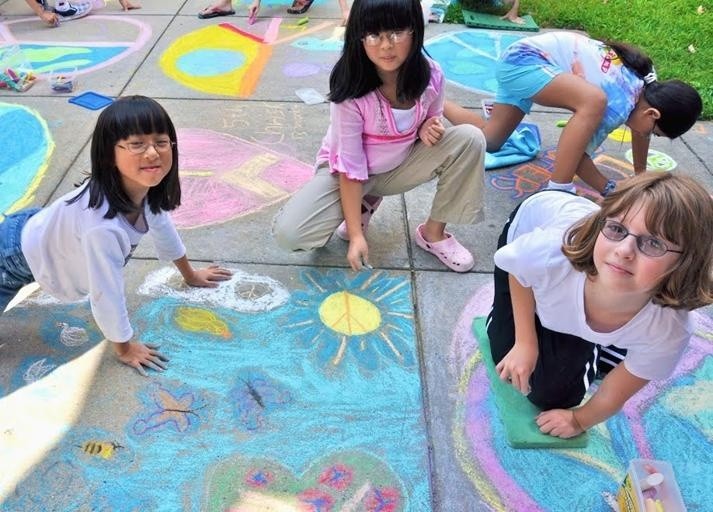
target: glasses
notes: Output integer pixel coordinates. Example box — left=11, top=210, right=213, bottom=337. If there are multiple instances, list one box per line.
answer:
left=360, top=30, right=415, bottom=44
left=596, top=218, right=687, bottom=257
left=115, top=137, right=177, bottom=153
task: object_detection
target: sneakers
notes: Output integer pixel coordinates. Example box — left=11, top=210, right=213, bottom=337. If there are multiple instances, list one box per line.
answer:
left=49, top=0, right=94, bottom=25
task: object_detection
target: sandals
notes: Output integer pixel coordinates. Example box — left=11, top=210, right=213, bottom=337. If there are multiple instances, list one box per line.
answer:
left=336, top=193, right=384, bottom=241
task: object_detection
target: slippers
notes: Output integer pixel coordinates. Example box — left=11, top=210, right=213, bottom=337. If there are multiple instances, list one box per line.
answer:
left=415, top=223, right=475, bottom=274
left=197, top=5, right=237, bottom=20
left=287, top=0, right=314, bottom=15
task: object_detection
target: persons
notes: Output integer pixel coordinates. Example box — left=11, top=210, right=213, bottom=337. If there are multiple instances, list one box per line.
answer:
left=271, top=1, right=486, bottom=275
left=441, top=31, right=703, bottom=196
left=499, top=1, right=525, bottom=25
left=25, top=0, right=142, bottom=26
left=0, top=95, right=234, bottom=375
left=486, top=173, right=713, bottom=439
left=198, top=1, right=349, bottom=26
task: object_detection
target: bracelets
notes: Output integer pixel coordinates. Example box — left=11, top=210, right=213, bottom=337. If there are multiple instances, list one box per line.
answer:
left=602, top=179, right=617, bottom=196
